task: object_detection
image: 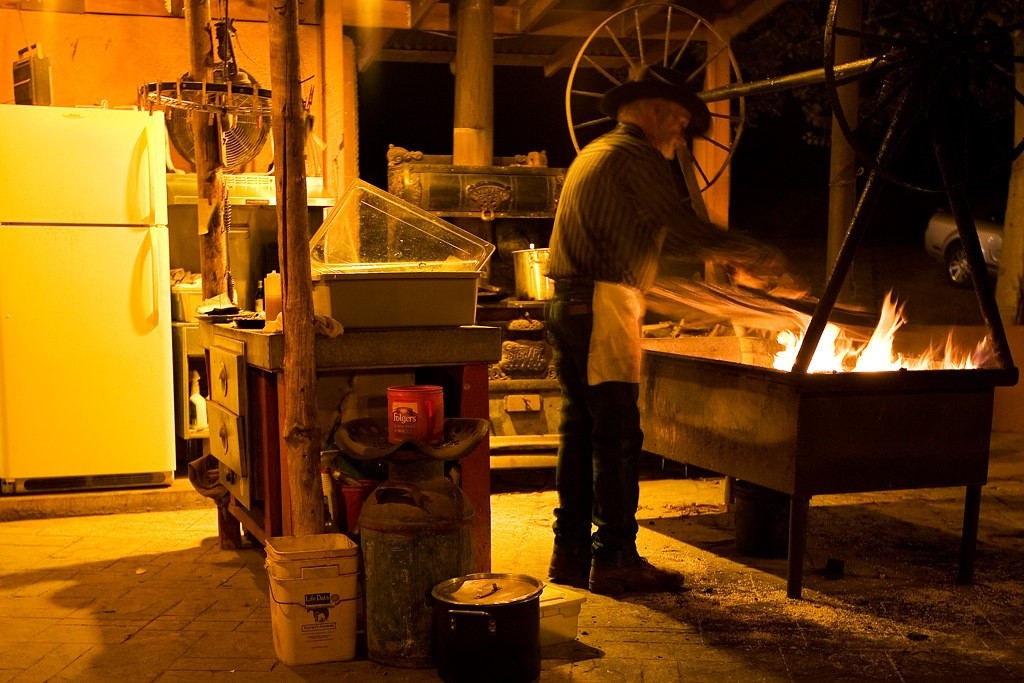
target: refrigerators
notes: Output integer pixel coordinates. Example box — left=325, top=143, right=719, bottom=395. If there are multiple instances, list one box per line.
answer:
left=0, top=104, right=176, bottom=491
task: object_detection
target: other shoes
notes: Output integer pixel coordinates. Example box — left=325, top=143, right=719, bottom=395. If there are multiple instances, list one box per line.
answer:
left=548, top=544, right=589, bottom=583
left=589, top=557, right=684, bottom=594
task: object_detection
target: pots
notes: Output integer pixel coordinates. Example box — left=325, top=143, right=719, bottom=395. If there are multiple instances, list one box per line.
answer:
left=512, top=248, right=555, bottom=301
left=431, top=572, right=547, bottom=683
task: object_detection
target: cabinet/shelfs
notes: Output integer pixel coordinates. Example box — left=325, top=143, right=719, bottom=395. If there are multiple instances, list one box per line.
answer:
left=197, top=324, right=503, bottom=574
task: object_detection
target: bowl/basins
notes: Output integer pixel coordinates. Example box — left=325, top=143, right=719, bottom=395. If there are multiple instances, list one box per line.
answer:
left=234, top=319, right=265, bottom=329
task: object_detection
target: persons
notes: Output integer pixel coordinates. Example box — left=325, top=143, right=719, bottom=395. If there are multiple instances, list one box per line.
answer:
left=547, top=63, right=813, bottom=595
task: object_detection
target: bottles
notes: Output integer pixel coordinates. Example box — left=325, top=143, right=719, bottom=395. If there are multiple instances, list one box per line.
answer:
left=320, top=467, right=334, bottom=525
left=255, top=280, right=263, bottom=312
left=263, top=270, right=281, bottom=322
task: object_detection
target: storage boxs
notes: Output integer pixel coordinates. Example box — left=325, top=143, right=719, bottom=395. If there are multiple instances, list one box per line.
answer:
left=540, top=584, right=587, bottom=647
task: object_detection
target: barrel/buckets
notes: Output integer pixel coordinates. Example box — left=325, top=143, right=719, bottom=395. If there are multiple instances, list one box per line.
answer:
left=388, top=385, right=444, bottom=446
left=334, top=479, right=378, bottom=535
left=264, top=533, right=358, bottom=664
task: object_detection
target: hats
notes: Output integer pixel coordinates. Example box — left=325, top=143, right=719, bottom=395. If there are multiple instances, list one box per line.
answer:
left=599, top=64, right=712, bottom=137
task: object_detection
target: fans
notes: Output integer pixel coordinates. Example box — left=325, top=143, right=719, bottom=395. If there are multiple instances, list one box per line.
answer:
left=165, top=67, right=268, bottom=170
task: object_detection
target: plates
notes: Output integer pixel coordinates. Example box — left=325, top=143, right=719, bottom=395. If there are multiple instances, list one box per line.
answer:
left=194, top=314, right=258, bottom=323
